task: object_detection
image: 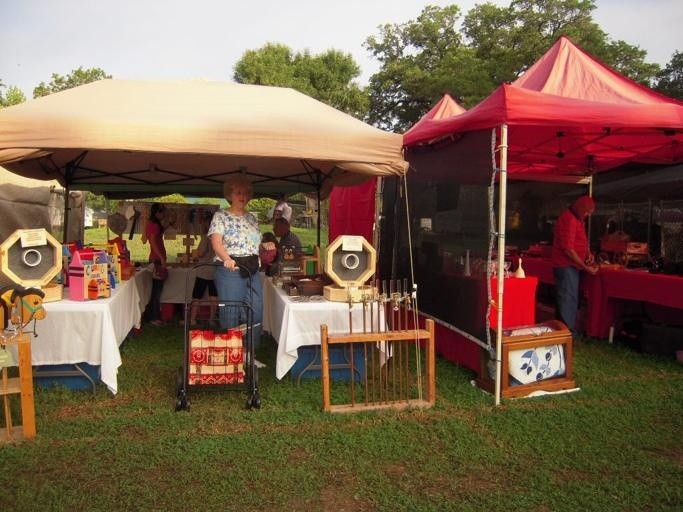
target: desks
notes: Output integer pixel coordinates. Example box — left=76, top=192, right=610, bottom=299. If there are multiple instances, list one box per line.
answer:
left=159, top=266, right=218, bottom=322
left=260, top=270, right=387, bottom=381
left=0, top=266, right=151, bottom=389
left=386, top=254, right=683, bottom=373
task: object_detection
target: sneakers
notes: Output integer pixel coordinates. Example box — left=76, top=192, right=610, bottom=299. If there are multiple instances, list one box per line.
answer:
left=251, top=358, right=267, bottom=369
left=177, top=319, right=199, bottom=328
left=143, top=319, right=167, bottom=329
left=206, top=318, right=218, bottom=327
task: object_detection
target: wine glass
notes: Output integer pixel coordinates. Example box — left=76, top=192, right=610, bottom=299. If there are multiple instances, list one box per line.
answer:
left=457, top=255, right=511, bottom=279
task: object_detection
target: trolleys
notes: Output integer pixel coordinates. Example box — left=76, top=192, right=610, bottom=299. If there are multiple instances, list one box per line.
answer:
left=176, top=262, right=265, bottom=411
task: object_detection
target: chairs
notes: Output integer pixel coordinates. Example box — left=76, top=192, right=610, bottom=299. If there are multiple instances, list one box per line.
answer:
left=294, top=246, right=321, bottom=277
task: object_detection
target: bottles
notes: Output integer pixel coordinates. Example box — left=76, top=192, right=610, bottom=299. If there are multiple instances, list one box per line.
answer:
left=514, top=258, right=526, bottom=278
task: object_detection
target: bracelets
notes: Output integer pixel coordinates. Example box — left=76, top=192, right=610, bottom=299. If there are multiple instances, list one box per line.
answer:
left=583, top=265, right=588, bottom=271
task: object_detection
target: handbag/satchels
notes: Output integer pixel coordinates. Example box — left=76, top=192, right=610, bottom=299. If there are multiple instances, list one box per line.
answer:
left=228, top=254, right=262, bottom=280
left=151, top=263, right=169, bottom=281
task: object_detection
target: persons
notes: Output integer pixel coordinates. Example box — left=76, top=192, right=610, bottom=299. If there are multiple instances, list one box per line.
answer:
left=272, top=193, right=293, bottom=223
left=274, top=218, right=303, bottom=261
left=261, top=233, right=277, bottom=245
left=203, top=173, right=275, bottom=370
left=548, top=195, right=601, bottom=348
left=141, top=200, right=167, bottom=325
left=187, top=210, right=221, bottom=328
